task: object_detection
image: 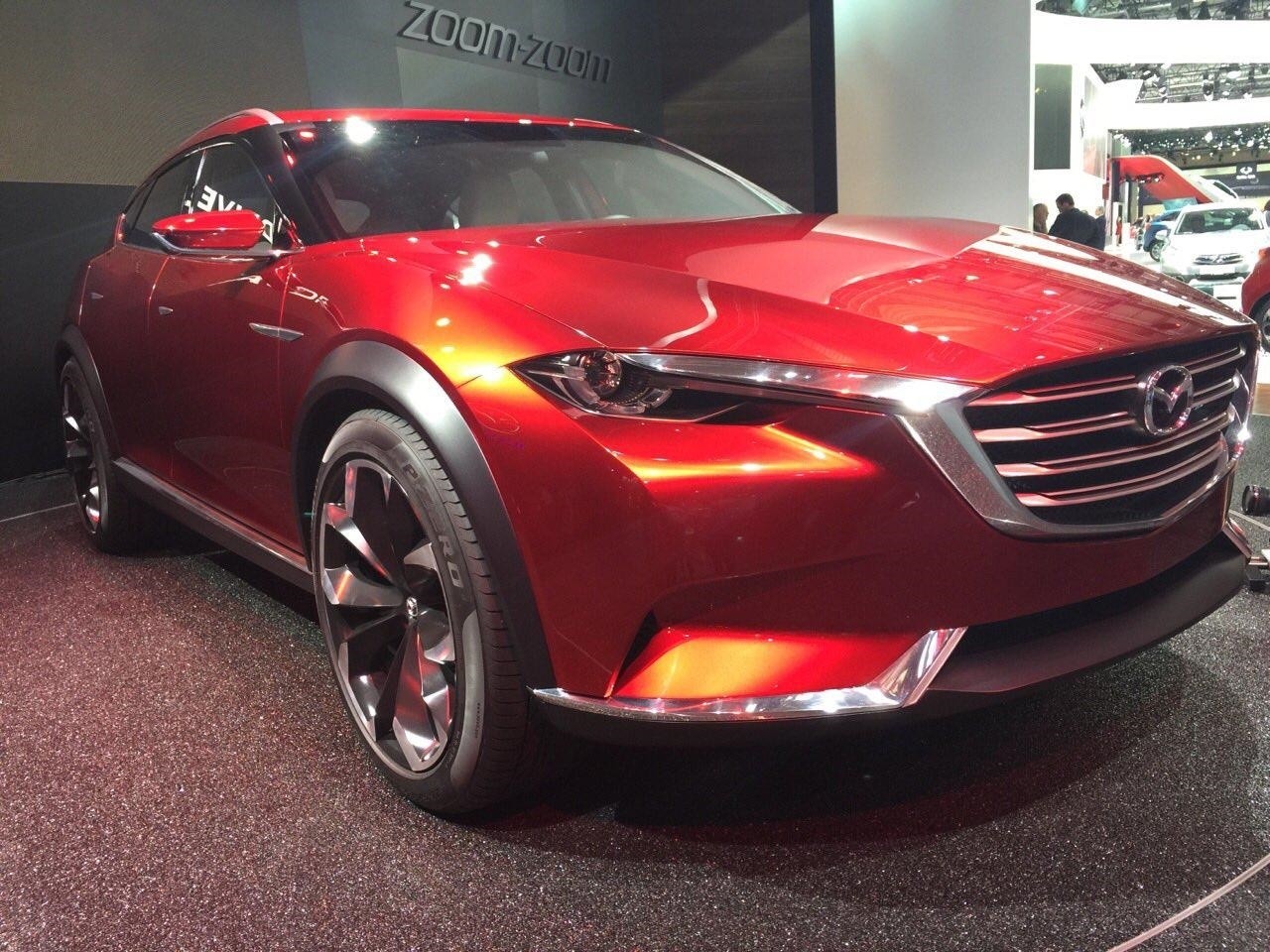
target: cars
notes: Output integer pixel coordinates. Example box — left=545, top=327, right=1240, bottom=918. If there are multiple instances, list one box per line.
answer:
left=1115, top=195, right=1270, bottom=352
left=60, top=108, right=1262, bottom=815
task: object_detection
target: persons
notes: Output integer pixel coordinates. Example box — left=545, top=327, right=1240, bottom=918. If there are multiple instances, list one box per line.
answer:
left=1094, top=205, right=1107, bottom=251
left=1047, top=194, right=1101, bottom=249
left=1033, top=203, right=1049, bottom=236
left=1115, top=215, right=1123, bottom=247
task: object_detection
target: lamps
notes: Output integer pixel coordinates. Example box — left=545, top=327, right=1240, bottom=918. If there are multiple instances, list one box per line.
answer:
left=1075, top=0, right=1270, bottom=165
